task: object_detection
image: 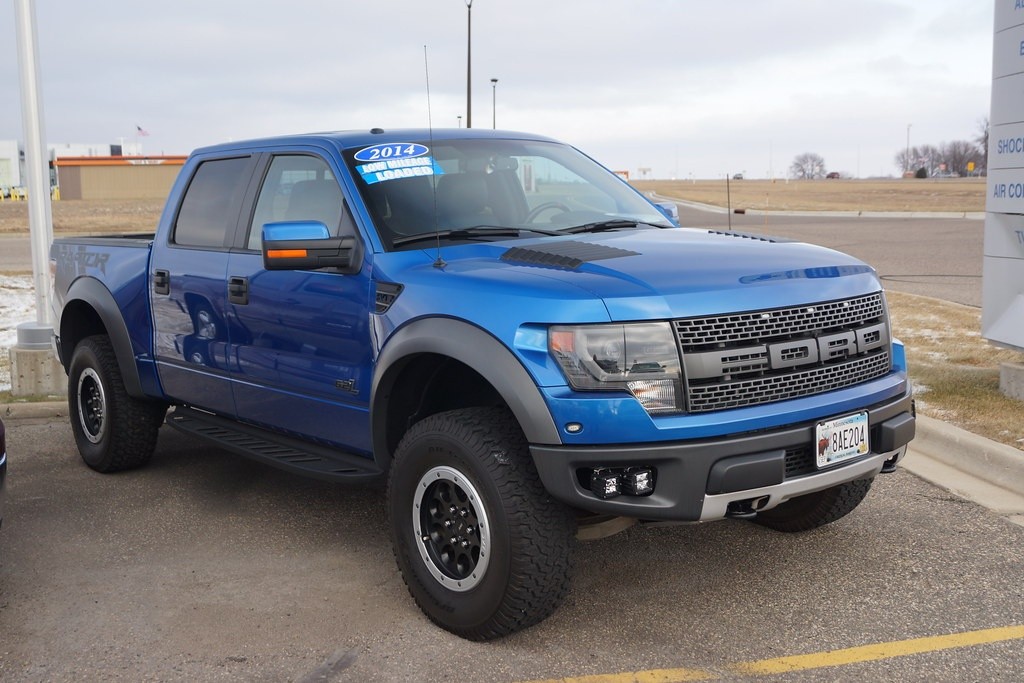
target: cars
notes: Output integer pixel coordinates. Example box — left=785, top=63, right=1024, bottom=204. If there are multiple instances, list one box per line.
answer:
left=827, top=172, right=839, bottom=178
left=733, top=174, right=743, bottom=179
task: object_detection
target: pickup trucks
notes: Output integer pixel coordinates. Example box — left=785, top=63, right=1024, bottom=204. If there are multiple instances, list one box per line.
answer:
left=47, top=126, right=919, bottom=643
